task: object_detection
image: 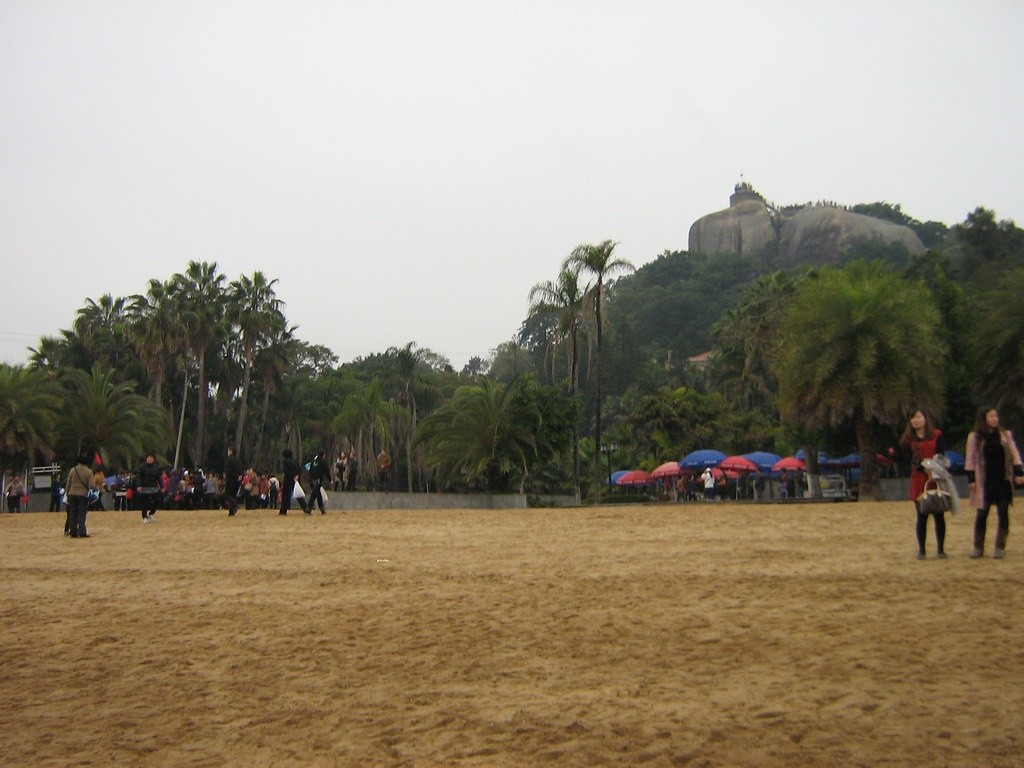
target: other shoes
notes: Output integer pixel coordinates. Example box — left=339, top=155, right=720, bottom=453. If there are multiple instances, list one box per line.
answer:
left=320, top=512, right=326, bottom=515
left=143, top=514, right=155, bottom=524
left=917, top=552, right=926, bottom=561
left=994, top=550, right=1005, bottom=558
left=70, top=533, right=91, bottom=538
left=64, top=532, right=70, bottom=536
left=229, top=505, right=239, bottom=516
left=304, top=511, right=311, bottom=515
left=937, top=551, right=947, bottom=559
left=969, top=548, right=983, bottom=558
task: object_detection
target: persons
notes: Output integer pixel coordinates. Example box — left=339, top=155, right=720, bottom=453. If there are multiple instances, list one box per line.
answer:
left=334, top=451, right=358, bottom=492
left=876, top=447, right=899, bottom=478
left=964, top=406, right=1024, bottom=558
left=89, top=468, right=141, bottom=511
left=677, top=468, right=727, bottom=502
left=736, top=467, right=805, bottom=499
left=6, top=474, right=24, bottom=513
left=225, top=447, right=244, bottom=516
left=136, top=450, right=165, bottom=524
left=49, top=473, right=60, bottom=512
left=900, top=407, right=949, bottom=559
left=279, top=448, right=333, bottom=515
left=159, top=466, right=229, bottom=510
left=377, top=449, right=391, bottom=492
left=243, top=468, right=282, bottom=510
left=63, top=454, right=95, bottom=538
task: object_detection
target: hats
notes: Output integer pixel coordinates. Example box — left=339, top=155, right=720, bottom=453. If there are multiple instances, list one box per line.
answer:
left=145, top=450, right=156, bottom=460
left=705, top=468, right=711, bottom=472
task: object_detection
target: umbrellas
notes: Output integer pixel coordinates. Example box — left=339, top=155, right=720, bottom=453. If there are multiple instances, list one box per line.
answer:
left=678, top=449, right=758, bottom=485
left=943, top=452, right=964, bottom=473
left=741, top=448, right=860, bottom=481
left=606, top=470, right=656, bottom=495
left=651, top=461, right=695, bottom=502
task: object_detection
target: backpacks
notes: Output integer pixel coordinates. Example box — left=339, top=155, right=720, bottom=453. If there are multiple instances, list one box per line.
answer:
left=270, top=481, right=278, bottom=496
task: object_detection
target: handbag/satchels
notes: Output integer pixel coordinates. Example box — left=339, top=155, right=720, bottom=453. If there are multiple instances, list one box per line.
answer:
left=320, top=487, right=328, bottom=503
left=1010, top=468, right=1024, bottom=496
left=718, top=476, right=726, bottom=487
left=293, top=481, right=305, bottom=499
left=245, top=483, right=253, bottom=491
left=87, top=488, right=99, bottom=504
left=915, top=479, right=953, bottom=514
